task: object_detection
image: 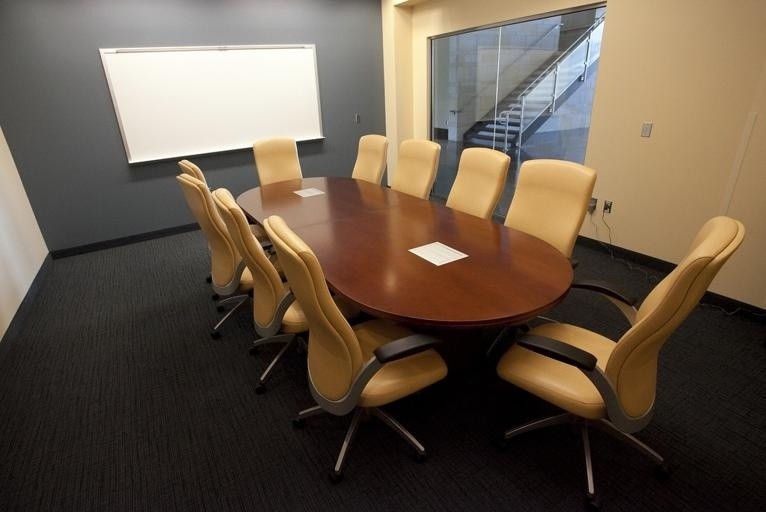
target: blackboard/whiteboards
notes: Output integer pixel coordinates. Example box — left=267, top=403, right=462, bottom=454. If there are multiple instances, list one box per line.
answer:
left=98, top=43, right=326, bottom=164
